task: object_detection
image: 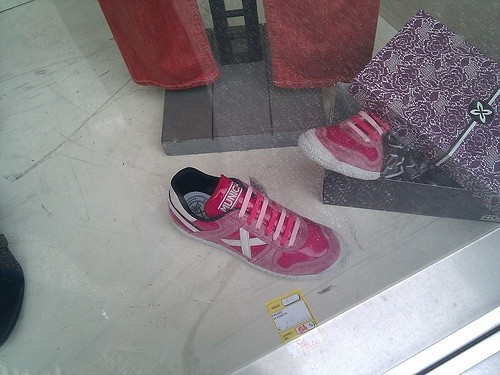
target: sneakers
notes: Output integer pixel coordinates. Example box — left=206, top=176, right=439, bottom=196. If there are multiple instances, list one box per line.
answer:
left=303, top=108, right=391, bottom=181
left=168, top=166, right=342, bottom=278
